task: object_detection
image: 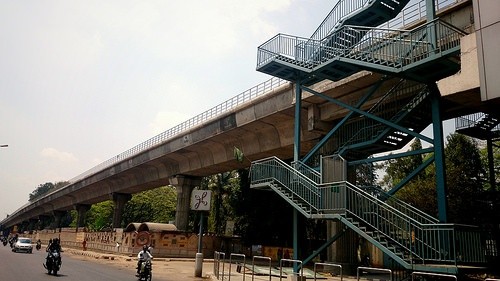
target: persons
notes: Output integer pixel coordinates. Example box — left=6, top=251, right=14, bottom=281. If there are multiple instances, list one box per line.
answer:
left=137, top=245, right=152, bottom=274
left=275, top=247, right=290, bottom=266
left=2, top=234, right=18, bottom=246
left=46, top=237, right=61, bottom=265
left=82, top=239, right=86, bottom=250
left=117, top=242, right=119, bottom=252
left=37, top=239, right=41, bottom=245
left=148, top=244, right=153, bottom=263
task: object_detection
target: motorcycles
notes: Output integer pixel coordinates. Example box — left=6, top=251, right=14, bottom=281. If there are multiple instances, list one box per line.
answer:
left=43, top=250, right=64, bottom=276
left=136, top=257, right=153, bottom=281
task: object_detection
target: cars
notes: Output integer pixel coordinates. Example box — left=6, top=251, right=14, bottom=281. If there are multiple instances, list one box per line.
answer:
left=13, top=237, right=33, bottom=253
